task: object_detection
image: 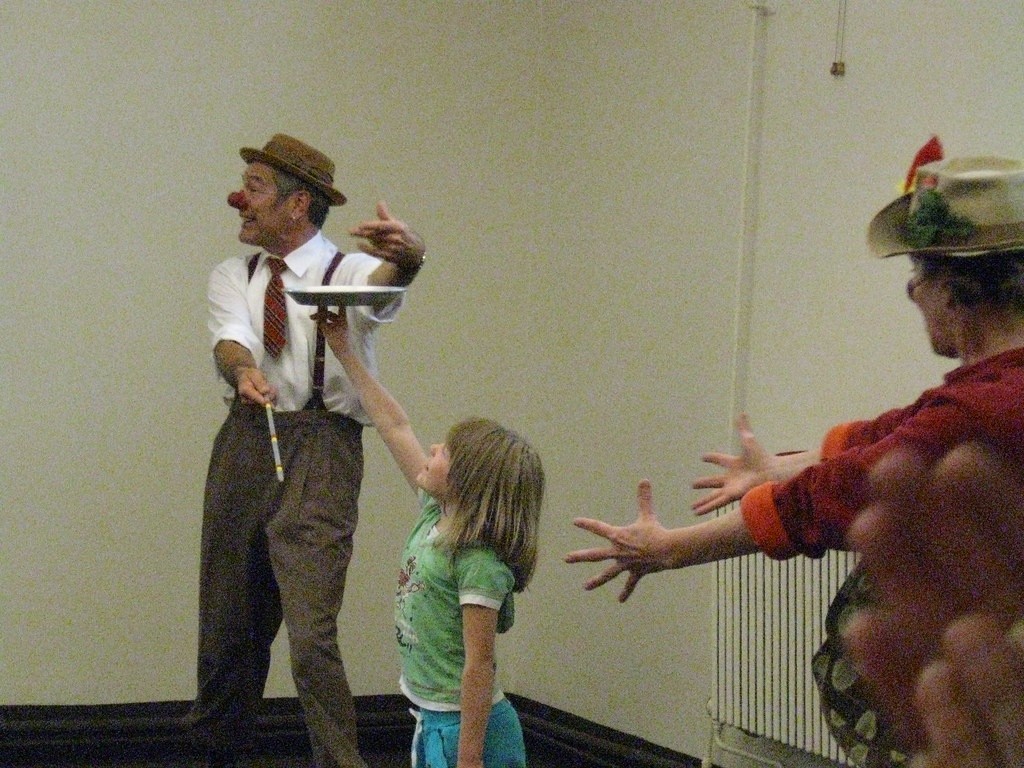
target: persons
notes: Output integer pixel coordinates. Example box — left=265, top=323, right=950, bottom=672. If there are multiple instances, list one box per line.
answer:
left=179, top=132, right=427, bottom=768
left=563, top=156, right=1024, bottom=768
left=309, top=303, right=546, bottom=768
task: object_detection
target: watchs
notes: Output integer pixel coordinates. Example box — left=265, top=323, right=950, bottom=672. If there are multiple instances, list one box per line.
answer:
left=397, top=251, right=427, bottom=276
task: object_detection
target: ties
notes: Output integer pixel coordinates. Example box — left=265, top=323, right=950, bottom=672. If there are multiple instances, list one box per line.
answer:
left=263, top=256, right=289, bottom=359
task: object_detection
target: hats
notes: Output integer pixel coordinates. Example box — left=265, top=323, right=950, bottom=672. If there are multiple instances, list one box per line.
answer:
left=863, top=153, right=1024, bottom=261
left=239, top=133, right=348, bottom=206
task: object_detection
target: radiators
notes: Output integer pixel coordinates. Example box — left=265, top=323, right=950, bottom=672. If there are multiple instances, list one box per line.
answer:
left=708, top=501, right=865, bottom=768
